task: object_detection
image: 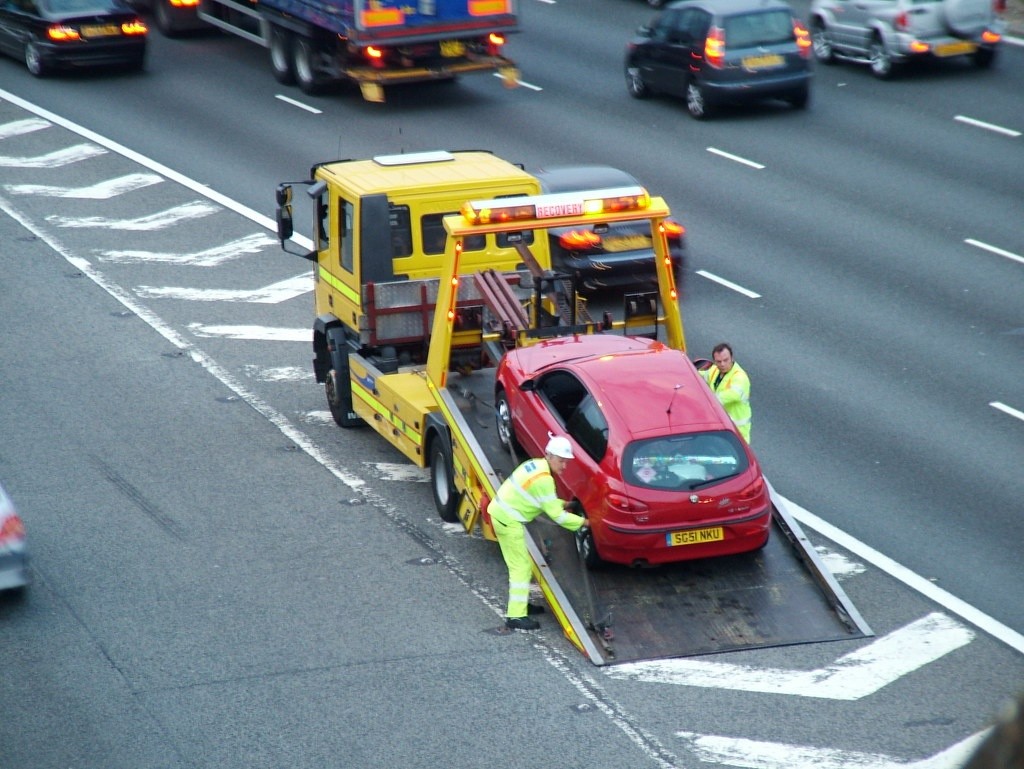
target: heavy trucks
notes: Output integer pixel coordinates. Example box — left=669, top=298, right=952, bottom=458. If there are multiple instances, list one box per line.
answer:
left=116, top=0, right=523, bottom=103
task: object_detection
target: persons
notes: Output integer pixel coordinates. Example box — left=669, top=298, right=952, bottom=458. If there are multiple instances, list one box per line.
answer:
left=698, top=344, right=752, bottom=446
left=487, top=436, right=590, bottom=630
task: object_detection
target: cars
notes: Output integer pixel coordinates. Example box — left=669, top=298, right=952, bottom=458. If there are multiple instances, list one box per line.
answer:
left=810, top=0, right=1006, bottom=81
left=531, top=164, right=686, bottom=299
left=0, top=0, right=149, bottom=77
left=0, top=487, right=37, bottom=591
left=623, top=0, right=816, bottom=120
left=495, top=333, right=774, bottom=573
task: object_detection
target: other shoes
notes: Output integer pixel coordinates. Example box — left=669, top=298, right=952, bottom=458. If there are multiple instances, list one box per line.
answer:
left=507, top=616, right=539, bottom=630
left=527, top=603, right=545, bottom=615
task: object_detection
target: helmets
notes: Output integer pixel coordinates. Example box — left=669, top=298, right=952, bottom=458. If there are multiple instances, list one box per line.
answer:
left=546, top=437, right=574, bottom=459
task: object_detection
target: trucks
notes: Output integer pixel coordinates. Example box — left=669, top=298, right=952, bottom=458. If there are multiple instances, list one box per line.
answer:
left=276, top=149, right=875, bottom=667
